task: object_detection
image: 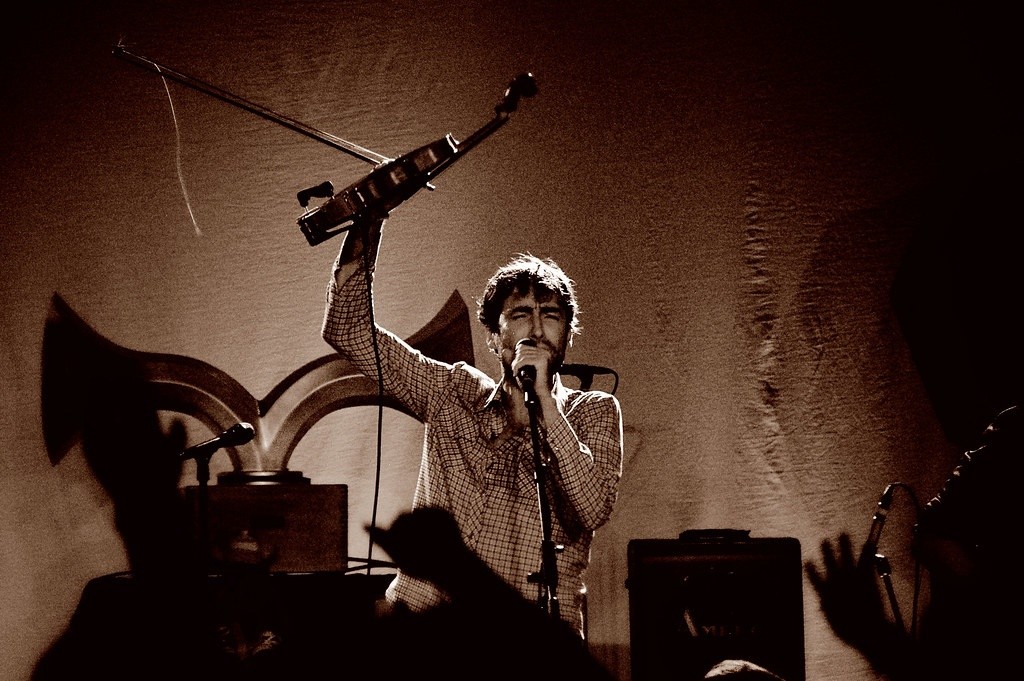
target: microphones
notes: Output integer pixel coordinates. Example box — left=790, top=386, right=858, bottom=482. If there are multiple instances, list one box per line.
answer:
left=859, top=485, right=897, bottom=564
left=516, top=337, right=537, bottom=385
left=179, top=422, right=255, bottom=462
left=559, top=364, right=613, bottom=375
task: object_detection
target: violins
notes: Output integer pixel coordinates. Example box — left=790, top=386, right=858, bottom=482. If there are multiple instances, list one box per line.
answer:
left=294, top=69, right=541, bottom=247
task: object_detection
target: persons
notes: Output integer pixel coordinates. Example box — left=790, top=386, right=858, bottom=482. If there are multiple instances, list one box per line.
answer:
left=321, top=157, right=622, bottom=641
left=28, top=371, right=910, bottom=681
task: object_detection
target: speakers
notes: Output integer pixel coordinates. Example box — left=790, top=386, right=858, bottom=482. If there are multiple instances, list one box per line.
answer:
left=627, top=536, right=806, bottom=681
